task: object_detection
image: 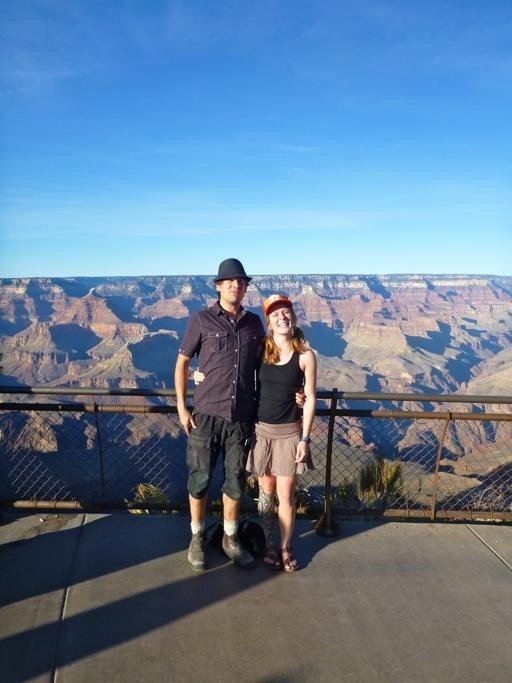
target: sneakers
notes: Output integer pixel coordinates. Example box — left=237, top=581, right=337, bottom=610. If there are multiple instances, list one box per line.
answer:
left=221, top=532, right=256, bottom=569
left=187, top=531, right=208, bottom=571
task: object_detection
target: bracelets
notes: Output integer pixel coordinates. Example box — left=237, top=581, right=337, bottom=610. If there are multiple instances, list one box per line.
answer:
left=300, top=435, right=310, bottom=444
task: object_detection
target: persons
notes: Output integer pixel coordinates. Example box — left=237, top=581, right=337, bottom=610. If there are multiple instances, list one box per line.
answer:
left=192, top=293, right=318, bottom=571
left=174, top=258, right=308, bottom=572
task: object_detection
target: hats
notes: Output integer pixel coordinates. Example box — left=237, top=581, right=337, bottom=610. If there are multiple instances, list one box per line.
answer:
left=261, top=294, right=292, bottom=316
left=213, top=258, right=252, bottom=282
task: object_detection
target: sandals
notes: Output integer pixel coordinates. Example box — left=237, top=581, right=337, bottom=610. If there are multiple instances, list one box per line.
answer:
left=280, top=547, right=299, bottom=573
